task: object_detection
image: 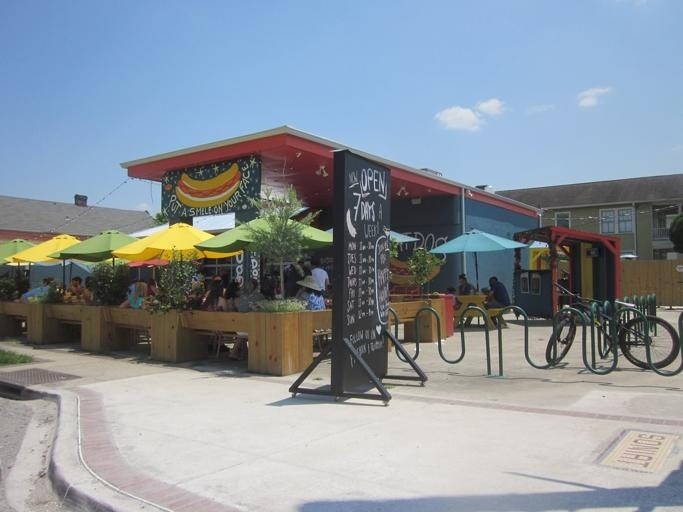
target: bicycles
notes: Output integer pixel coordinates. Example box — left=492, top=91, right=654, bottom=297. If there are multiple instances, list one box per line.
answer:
left=545, top=280, right=680, bottom=370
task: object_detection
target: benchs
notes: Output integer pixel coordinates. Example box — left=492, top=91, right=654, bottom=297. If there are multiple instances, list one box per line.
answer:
left=451, top=305, right=511, bottom=328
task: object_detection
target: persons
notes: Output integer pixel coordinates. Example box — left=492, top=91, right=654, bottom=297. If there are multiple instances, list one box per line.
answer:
left=458, top=277, right=474, bottom=296
left=40, top=260, right=330, bottom=352
left=479, top=277, right=511, bottom=328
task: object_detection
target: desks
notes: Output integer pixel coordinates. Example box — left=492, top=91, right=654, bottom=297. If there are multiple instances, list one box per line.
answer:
left=460, top=291, right=486, bottom=309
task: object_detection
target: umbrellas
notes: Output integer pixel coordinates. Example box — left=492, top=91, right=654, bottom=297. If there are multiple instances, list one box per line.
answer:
left=428, top=227, right=528, bottom=293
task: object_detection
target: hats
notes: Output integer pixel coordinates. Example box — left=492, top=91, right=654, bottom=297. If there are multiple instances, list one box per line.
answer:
left=295, top=275, right=322, bottom=292
